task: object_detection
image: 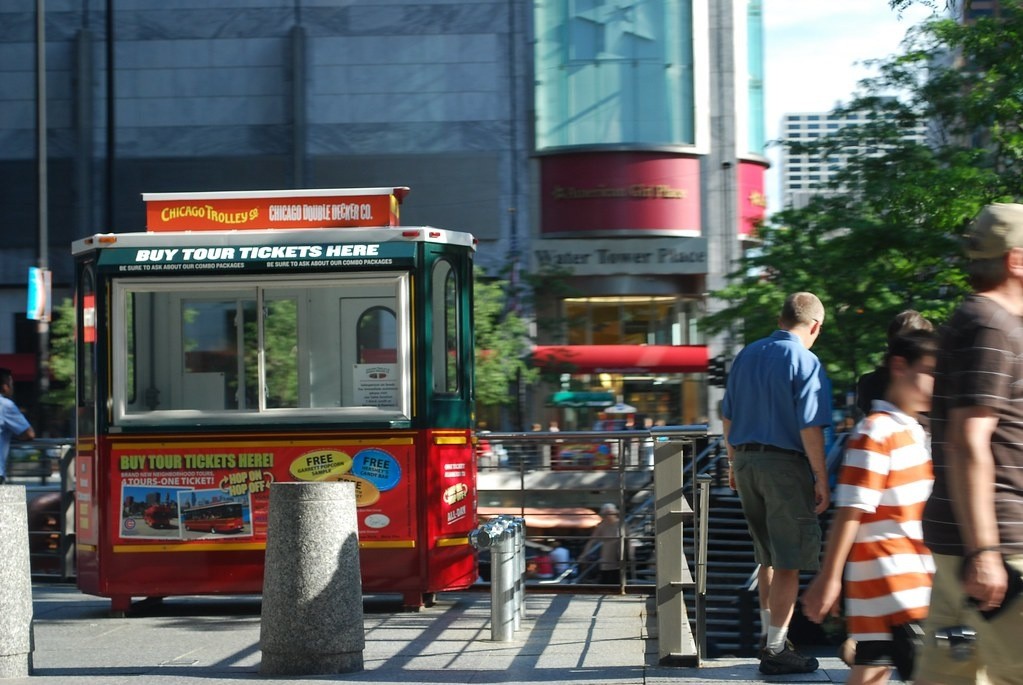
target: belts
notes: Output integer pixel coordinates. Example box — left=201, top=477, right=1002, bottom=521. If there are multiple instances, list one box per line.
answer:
left=735, top=443, right=797, bottom=454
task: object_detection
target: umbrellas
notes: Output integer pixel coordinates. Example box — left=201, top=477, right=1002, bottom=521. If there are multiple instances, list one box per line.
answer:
left=605, top=404, right=636, bottom=414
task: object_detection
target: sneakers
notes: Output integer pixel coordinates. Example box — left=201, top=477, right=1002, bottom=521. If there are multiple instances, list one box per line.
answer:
left=757, top=634, right=805, bottom=658
left=759, top=645, right=819, bottom=674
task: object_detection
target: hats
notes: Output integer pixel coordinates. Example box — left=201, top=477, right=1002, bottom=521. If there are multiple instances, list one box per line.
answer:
left=600, top=502, right=618, bottom=514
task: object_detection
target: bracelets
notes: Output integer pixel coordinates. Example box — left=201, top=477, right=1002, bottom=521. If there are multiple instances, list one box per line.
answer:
left=962, top=545, right=997, bottom=561
left=728, top=459, right=734, bottom=466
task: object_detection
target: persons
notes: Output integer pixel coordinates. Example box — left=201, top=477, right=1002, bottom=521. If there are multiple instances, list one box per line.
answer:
left=531, top=423, right=540, bottom=431
left=888, top=310, right=933, bottom=345
left=923, top=201, right=1021, bottom=685
left=800, top=329, right=939, bottom=684
left=550, top=422, right=565, bottom=443
left=532, top=541, right=570, bottom=580
left=722, top=292, right=830, bottom=673
left=0, top=367, right=35, bottom=484
left=578, top=503, right=625, bottom=591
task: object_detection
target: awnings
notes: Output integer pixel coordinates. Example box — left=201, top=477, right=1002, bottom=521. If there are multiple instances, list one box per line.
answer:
left=532, top=345, right=708, bottom=372
left=547, top=390, right=615, bottom=407
